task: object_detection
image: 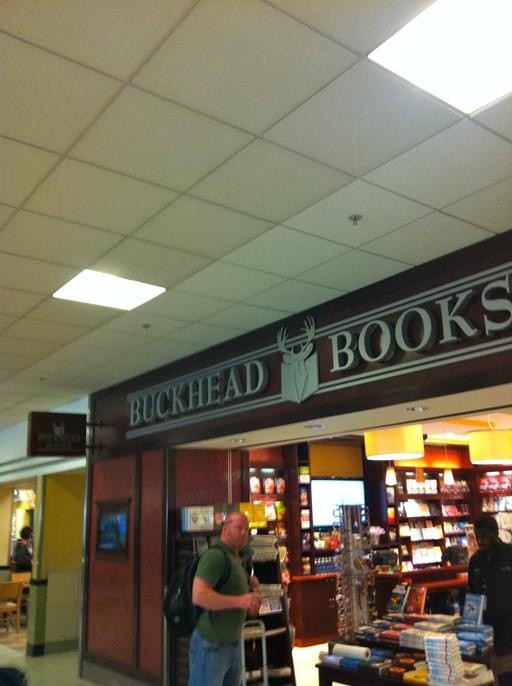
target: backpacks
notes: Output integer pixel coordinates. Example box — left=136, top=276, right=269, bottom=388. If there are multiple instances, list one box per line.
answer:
left=161, top=543, right=233, bottom=637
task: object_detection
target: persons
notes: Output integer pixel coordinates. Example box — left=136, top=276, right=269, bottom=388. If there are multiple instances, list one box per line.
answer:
left=13, top=526, right=34, bottom=614
left=186, top=511, right=265, bottom=686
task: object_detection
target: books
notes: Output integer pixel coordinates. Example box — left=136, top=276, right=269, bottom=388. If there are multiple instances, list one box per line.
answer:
left=180, top=504, right=285, bottom=617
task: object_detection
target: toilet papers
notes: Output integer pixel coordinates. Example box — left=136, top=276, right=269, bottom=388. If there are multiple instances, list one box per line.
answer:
left=332, top=643, right=371, bottom=661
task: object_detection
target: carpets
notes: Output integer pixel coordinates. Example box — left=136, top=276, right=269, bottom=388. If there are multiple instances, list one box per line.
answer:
left=1, top=629, right=27, bottom=652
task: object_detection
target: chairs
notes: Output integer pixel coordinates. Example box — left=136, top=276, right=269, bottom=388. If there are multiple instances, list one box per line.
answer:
left=0, top=572, right=32, bottom=636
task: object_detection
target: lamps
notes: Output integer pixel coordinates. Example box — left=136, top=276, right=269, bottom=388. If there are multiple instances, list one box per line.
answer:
left=365, top=422, right=512, bottom=466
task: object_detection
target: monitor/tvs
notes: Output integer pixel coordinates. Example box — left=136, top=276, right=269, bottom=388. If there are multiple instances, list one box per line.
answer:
left=423, top=585, right=456, bottom=614
left=309, top=475, right=368, bottom=529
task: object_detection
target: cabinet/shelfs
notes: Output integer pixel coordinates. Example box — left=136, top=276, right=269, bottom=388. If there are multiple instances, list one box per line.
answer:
left=172, top=443, right=512, bottom=686
